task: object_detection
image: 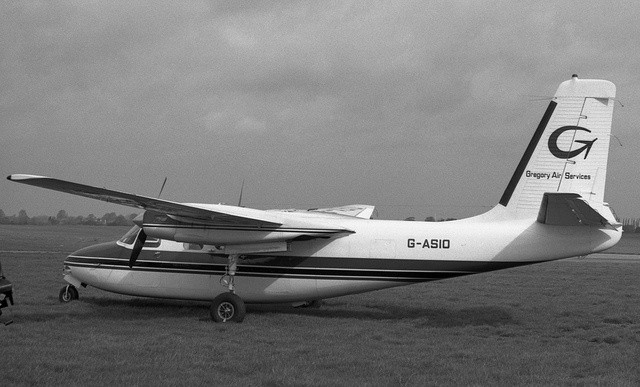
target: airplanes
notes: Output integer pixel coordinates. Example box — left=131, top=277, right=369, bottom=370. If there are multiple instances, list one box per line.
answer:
left=9, top=75, right=623, bottom=323
